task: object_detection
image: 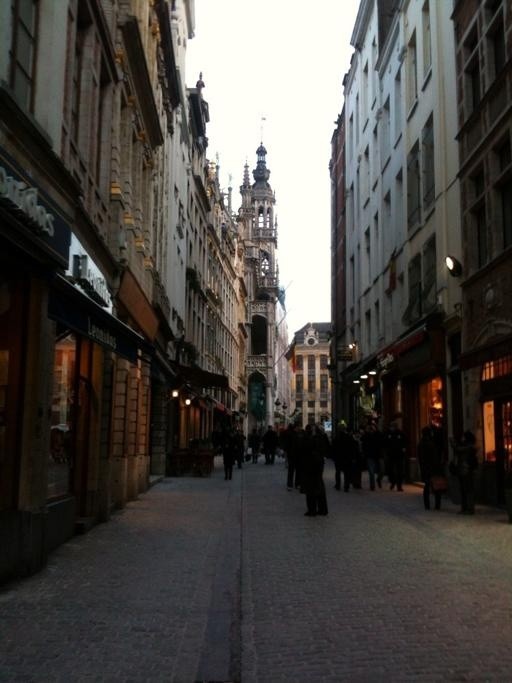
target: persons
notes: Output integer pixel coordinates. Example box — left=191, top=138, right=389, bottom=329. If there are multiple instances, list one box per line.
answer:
left=221, top=422, right=407, bottom=493
left=416, top=426, right=480, bottom=515
left=297, top=424, right=332, bottom=517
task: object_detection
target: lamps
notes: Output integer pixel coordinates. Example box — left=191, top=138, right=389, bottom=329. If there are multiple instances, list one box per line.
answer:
left=184, top=394, right=196, bottom=407
left=169, top=389, right=179, bottom=400
left=360, top=373, right=368, bottom=379
left=352, top=379, right=360, bottom=384
left=349, top=342, right=356, bottom=349
left=369, top=369, right=377, bottom=375
left=445, top=255, right=461, bottom=276
